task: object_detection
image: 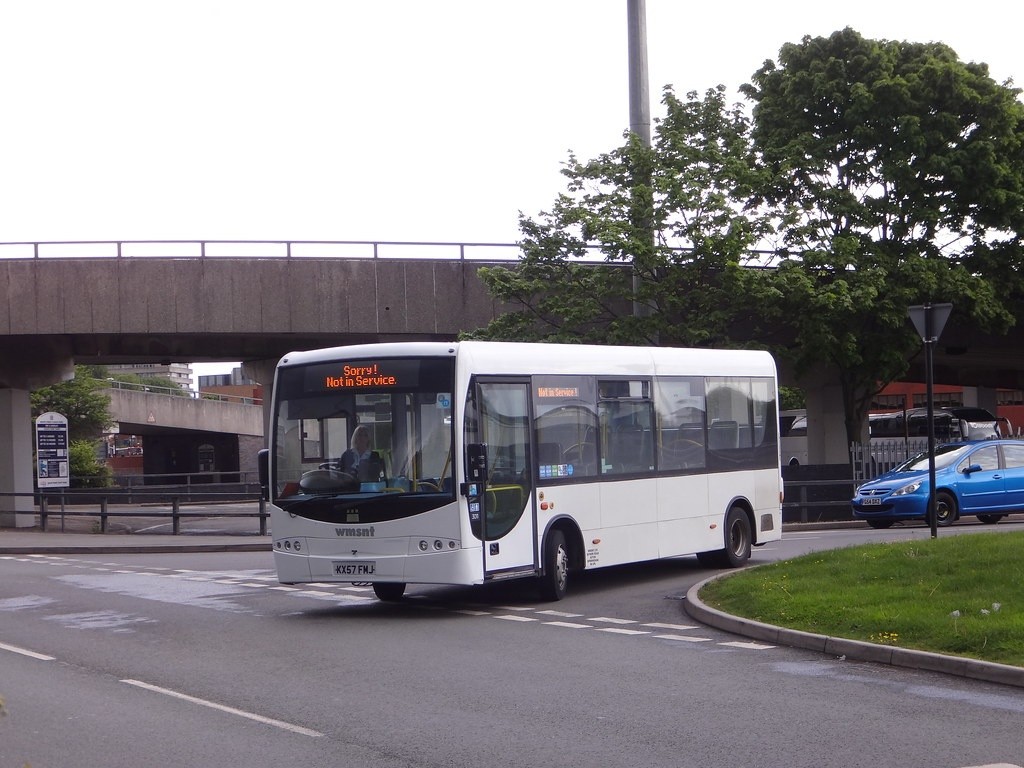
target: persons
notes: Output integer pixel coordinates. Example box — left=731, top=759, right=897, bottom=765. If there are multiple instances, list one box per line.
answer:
left=339, top=427, right=387, bottom=489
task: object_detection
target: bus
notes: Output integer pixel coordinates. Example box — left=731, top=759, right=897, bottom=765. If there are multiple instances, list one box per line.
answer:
left=257, top=341, right=786, bottom=609
left=776, top=406, right=1014, bottom=469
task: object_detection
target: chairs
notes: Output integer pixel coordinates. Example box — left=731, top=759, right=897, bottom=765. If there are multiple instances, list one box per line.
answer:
left=578, top=417, right=740, bottom=472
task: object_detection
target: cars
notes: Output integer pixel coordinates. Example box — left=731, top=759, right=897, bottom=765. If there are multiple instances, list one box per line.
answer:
left=850, top=437, right=1024, bottom=530
